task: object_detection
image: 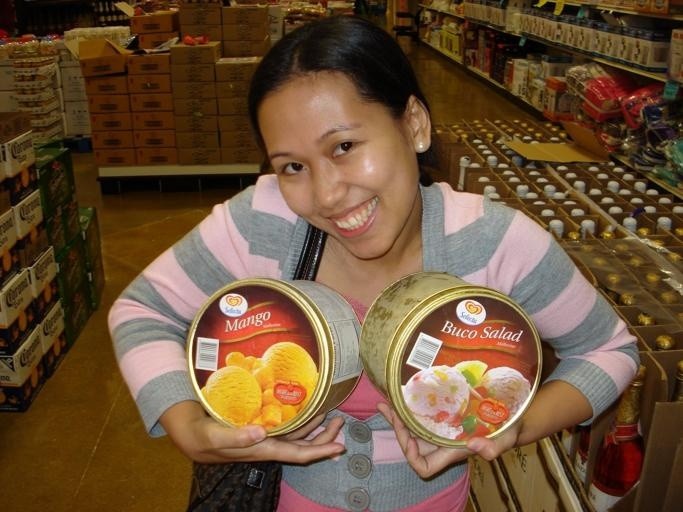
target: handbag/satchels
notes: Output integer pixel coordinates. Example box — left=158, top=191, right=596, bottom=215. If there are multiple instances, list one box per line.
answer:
left=186, top=225, right=327, bottom=512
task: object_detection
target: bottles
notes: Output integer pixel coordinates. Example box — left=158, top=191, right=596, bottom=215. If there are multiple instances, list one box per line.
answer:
left=44, top=169, right=85, bottom=325
left=559, top=229, right=681, bottom=512
left=430, top=115, right=683, bottom=242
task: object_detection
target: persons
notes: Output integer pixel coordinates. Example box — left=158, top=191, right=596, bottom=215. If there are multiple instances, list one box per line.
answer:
left=105, top=11, right=643, bottom=512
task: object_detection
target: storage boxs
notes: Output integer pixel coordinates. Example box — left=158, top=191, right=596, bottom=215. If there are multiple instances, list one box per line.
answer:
left=0, top=40, right=92, bottom=144
left=421, top=115, right=682, bottom=511
left=0, top=109, right=106, bottom=413
left=77, top=0, right=283, bottom=164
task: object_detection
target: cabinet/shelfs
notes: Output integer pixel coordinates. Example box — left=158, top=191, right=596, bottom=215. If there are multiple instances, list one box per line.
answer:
left=392, top=0, right=412, bottom=30
left=412, top=0, right=682, bottom=122
